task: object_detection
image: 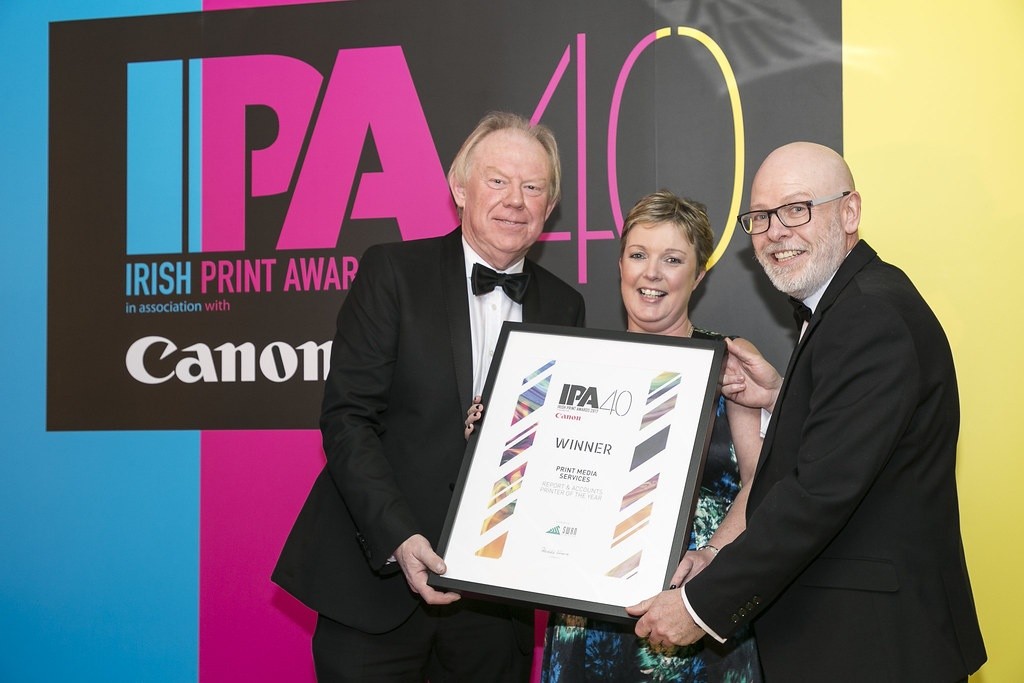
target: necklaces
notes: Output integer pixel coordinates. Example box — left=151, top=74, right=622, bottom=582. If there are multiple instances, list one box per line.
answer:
left=687, top=325, right=694, bottom=338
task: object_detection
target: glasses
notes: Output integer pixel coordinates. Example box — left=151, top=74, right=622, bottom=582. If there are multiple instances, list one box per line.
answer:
left=737, top=190, right=852, bottom=236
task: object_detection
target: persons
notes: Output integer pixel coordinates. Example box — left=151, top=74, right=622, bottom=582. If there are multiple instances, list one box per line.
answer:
left=463, top=189, right=769, bottom=682
left=272, top=109, right=587, bottom=683
left=626, top=141, right=987, bottom=683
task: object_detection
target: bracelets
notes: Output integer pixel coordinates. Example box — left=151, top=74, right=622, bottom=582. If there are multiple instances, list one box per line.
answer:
left=698, top=544, right=718, bottom=557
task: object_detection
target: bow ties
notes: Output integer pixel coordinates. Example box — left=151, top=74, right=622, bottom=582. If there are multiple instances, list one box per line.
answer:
left=788, top=296, right=813, bottom=331
left=471, top=263, right=531, bottom=304
